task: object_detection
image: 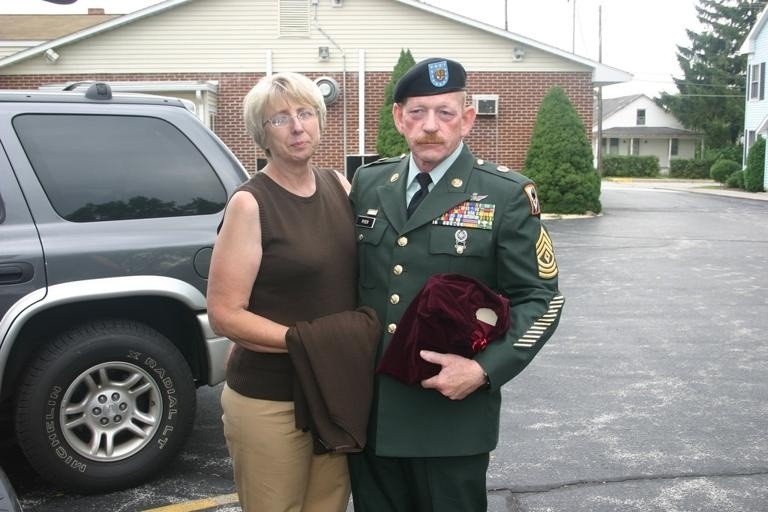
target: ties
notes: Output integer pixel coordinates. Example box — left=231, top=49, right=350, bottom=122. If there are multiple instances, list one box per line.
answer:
left=406, top=172, right=432, bottom=220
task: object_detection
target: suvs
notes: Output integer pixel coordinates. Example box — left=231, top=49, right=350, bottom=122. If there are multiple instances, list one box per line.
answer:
left=0, top=80, right=254, bottom=497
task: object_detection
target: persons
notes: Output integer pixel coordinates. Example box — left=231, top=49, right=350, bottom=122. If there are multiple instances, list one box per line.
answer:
left=346, top=57, right=564, bottom=511
left=205, top=69, right=362, bottom=511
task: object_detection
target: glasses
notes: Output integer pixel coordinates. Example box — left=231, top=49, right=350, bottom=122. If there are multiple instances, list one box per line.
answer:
left=262, top=110, right=318, bottom=130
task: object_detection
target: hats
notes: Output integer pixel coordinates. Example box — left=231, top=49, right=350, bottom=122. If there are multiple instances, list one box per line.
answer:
left=394, top=58, right=467, bottom=104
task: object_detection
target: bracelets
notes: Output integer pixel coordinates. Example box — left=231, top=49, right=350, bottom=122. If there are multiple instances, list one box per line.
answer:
left=483, top=371, right=491, bottom=386
